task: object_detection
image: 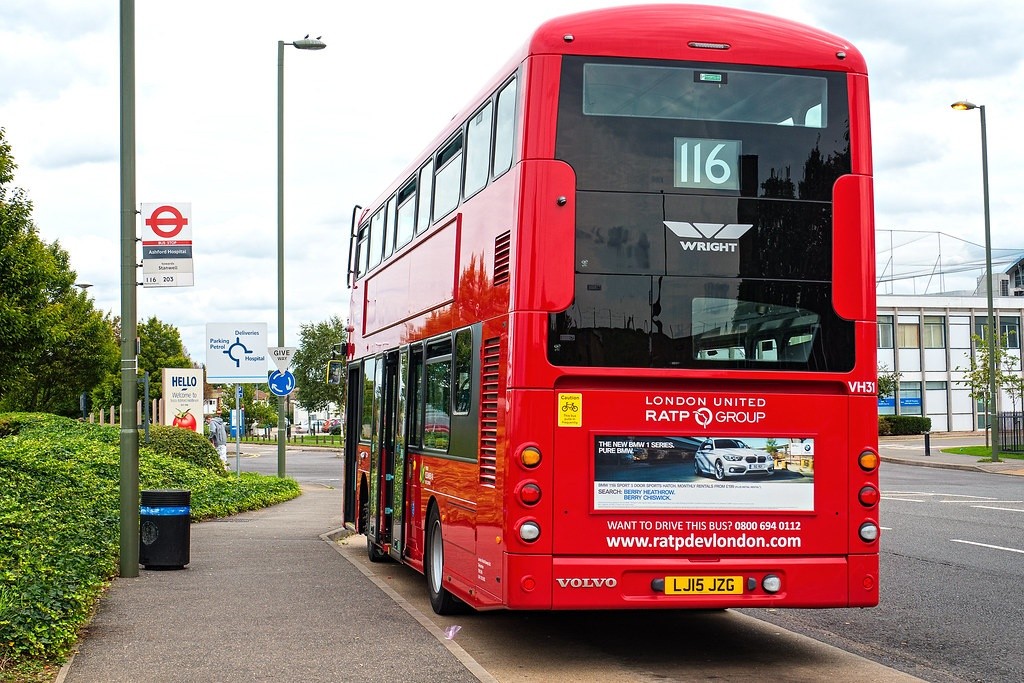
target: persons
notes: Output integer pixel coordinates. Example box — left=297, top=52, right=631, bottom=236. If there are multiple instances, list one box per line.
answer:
left=205, top=414, right=227, bottom=472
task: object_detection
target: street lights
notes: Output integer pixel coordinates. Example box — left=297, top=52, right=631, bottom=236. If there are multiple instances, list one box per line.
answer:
left=952, top=101, right=1002, bottom=463
left=275, top=35, right=327, bottom=477
left=73, top=284, right=94, bottom=297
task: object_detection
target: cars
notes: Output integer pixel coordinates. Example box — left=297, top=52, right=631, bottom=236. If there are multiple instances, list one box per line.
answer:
left=294, top=419, right=342, bottom=435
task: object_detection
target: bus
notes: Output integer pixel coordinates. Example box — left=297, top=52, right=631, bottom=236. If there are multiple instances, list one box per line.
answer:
left=324, top=2, right=885, bottom=612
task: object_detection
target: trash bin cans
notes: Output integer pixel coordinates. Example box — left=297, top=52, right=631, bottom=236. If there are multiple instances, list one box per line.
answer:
left=136, top=488, right=192, bottom=570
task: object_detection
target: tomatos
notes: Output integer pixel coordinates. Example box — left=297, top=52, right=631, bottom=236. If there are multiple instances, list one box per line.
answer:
left=172, top=408, right=196, bottom=431
